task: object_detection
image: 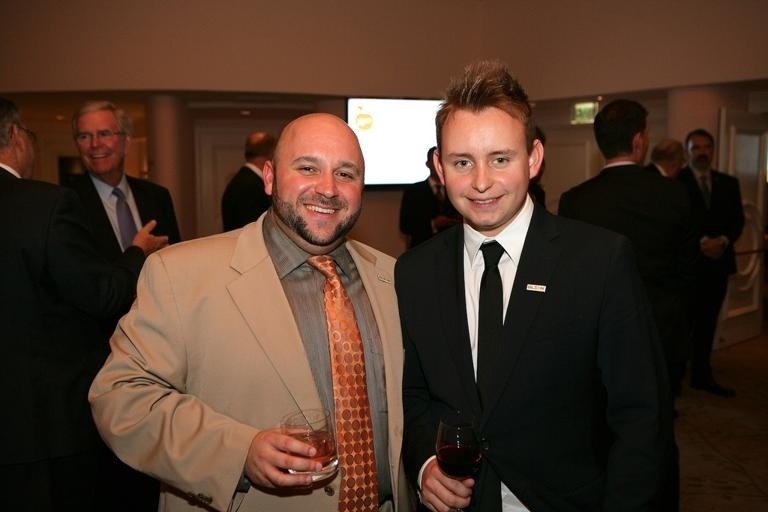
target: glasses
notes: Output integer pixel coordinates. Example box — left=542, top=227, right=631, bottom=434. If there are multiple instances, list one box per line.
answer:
left=74, top=130, right=123, bottom=144
left=16, top=126, right=36, bottom=143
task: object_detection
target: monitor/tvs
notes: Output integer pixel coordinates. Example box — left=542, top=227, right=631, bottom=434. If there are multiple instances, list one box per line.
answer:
left=345, top=96, right=445, bottom=188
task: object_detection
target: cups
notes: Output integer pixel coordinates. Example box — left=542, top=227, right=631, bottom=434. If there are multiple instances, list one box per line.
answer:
left=279, top=408, right=338, bottom=483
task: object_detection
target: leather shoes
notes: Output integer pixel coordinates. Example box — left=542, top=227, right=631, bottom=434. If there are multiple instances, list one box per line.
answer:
left=690, top=378, right=735, bottom=397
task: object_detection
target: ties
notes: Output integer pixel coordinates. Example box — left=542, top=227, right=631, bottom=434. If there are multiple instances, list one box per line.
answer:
left=306, top=255, right=379, bottom=511
left=435, top=185, right=442, bottom=201
left=476, top=241, right=503, bottom=511
left=113, top=188, right=138, bottom=251
left=699, top=175, right=710, bottom=208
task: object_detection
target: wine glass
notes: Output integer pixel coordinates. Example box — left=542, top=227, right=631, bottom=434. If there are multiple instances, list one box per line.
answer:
left=435, top=409, right=483, bottom=512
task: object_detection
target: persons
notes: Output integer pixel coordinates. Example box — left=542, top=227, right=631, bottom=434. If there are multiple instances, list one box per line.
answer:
left=394, top=61, right=680, bottom=512
left=58, top=101, right=184, bottom=512
left=222, top=131, right=277, bottom=232
left=88, top=112, right=420, bottom=512
left=0, top=97, right=170, bottom=512
